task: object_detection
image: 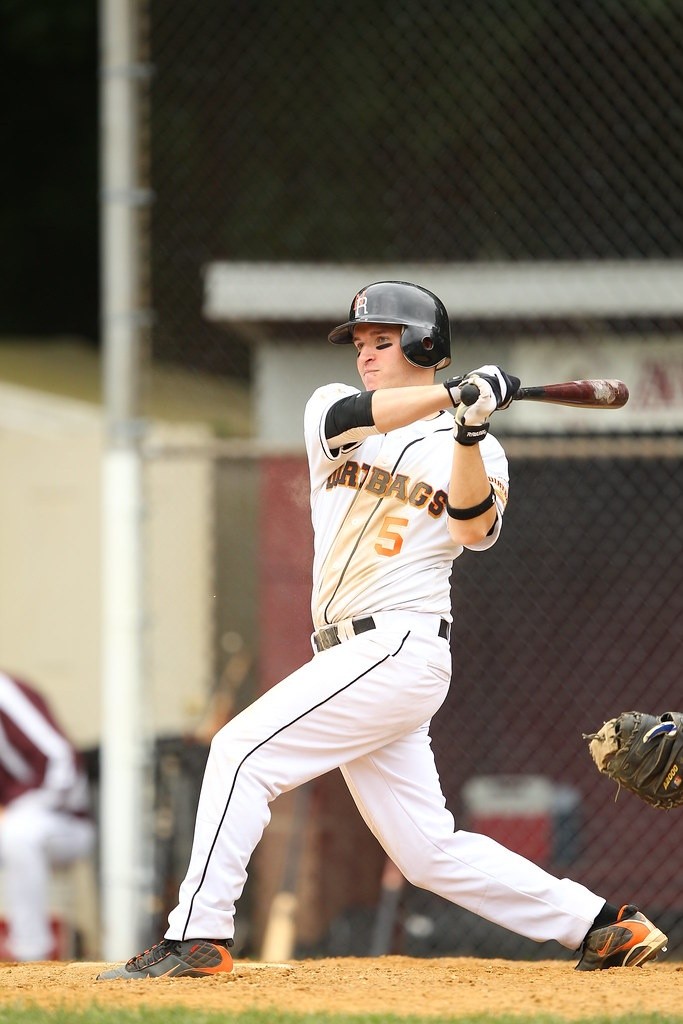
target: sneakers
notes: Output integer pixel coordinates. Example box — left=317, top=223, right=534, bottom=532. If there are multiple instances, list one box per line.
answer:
left=572, top=906, right=668, bottom=972
left=95, top=935, right=234, bottom=982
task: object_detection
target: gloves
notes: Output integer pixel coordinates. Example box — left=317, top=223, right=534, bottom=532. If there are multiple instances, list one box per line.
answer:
left=444, top=365, right=521, bottom=411
left=458, top=376, right=502, bottom=446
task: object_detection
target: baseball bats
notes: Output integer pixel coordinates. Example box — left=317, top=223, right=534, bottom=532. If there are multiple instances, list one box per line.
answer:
left=461, top=378, right=629, bottom=410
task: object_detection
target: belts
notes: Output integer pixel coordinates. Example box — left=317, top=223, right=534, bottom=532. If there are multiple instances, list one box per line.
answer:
left=314, top=615, right=449, bottom=653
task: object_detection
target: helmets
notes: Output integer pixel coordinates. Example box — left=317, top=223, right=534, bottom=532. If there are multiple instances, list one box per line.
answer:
left=328, top=281, right=452, bottom=371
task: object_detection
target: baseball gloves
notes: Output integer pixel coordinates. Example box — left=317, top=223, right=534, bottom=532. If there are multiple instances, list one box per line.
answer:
left=581, top=710, right=683, bottom=812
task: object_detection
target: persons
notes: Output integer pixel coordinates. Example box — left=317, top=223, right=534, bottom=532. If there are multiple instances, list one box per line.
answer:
left=0, top=668, right=96, bottom=962
left=94, top=281, right=669, bottom=982
